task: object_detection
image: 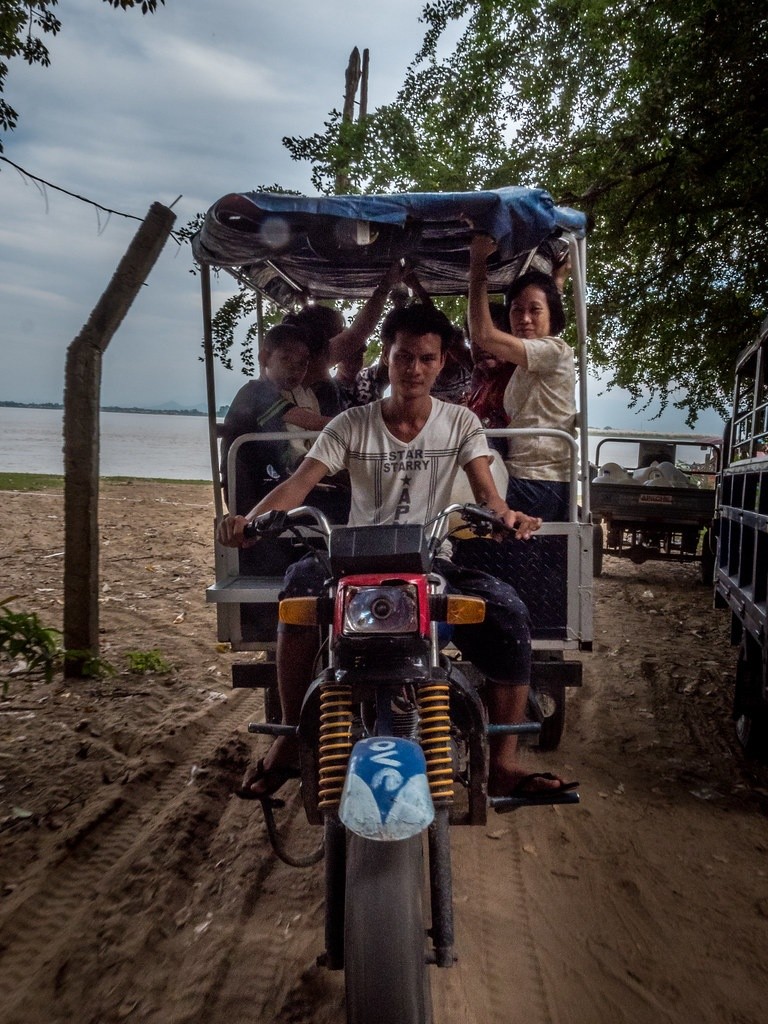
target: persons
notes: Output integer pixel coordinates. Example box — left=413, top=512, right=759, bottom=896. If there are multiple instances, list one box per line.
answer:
left=216, top=304, right=580, bottom=805
left=216, top=236, right=577, bottom=523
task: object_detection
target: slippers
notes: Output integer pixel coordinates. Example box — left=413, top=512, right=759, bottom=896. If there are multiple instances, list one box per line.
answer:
left=492, top=771, right=581, bottom=812
left=234, top=760, right=301, bottom=800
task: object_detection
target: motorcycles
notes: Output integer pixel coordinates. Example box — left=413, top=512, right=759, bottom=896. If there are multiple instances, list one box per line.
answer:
left=190, top=186, right=597, bottom=1024
left=588, top=437, right=721, bottom=587
